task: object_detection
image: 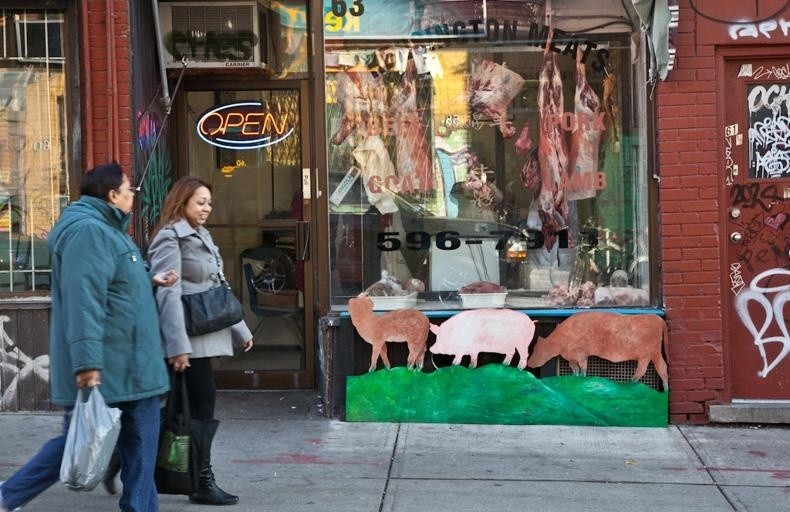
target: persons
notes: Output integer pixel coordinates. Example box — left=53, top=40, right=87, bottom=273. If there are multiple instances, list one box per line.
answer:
left=0, top=159, right=180, bottom=512
left=450, top=157, right=505, bottom=222
left=354, top=192, right=430, bottom=298
left=511, top=148, right=560, bottom=267
left=146, top=178, right=254, bottom=506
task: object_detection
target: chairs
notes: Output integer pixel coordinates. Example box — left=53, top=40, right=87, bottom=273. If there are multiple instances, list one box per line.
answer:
left=240, top=245, right=304, bottom=354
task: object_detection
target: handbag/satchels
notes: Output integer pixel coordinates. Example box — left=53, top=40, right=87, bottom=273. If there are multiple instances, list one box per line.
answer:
left=150, top=363, right=219, bottom=496
left=181, top=284, right=246, bottom=338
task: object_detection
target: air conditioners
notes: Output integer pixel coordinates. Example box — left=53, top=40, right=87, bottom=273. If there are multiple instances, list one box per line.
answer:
left=157, top=1, right=277, bottom=73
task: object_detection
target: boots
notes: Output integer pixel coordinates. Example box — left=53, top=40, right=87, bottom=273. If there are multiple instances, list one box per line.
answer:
left=185, top=419, right=242, bottom=505
left=102, top=453, right=123, bottom=497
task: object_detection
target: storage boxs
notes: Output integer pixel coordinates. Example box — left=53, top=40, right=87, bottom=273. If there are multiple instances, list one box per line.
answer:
left=358, top=292, right=418, bottom=311
left=457, top=287, right=510, bottom=308
